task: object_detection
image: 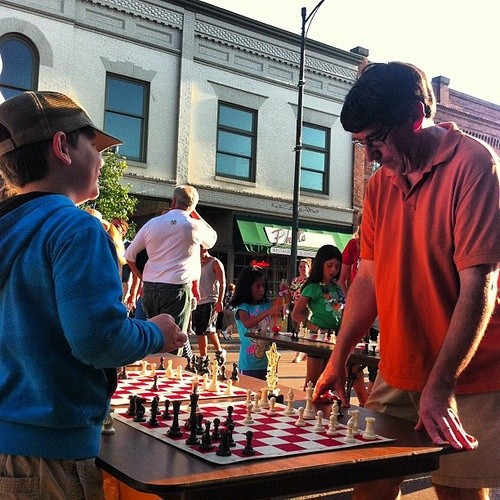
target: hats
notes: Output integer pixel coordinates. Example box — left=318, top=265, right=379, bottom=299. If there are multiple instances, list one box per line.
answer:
left=85, top=208, right=110, bottom=233
left=0, top=90, right=124, bottom=157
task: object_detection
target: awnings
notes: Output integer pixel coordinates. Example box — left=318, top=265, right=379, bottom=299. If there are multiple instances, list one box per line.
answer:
left=234, top=215, right=357, bottom=262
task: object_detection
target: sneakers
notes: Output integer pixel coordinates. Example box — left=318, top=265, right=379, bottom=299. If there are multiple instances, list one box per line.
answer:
left=215, top=349, right=226, bottom=367
left=197, top=355, right=210, bottom=367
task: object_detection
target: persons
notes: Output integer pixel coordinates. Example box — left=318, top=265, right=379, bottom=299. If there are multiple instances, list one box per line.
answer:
left=304, top=62, right=500, bottom=499
left=284, top=259, right=315, bottom=363
left=83, top=206, right=148, bottom=330
left=338, top=220, right=382, bottom=409
left=123, top=184, right=238, bottom=367
left=230, top=263, right=291, bottom=382
left=1, top=92, right=189, bottom=500
left=291, top=244, right=351, bottom=407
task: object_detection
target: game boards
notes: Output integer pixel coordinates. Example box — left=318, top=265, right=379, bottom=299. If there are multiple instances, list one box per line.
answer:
left=280, top=332, right=339, bottom=344
left=109, top=368, right=258, bottom=407
left=354, top=343, right=381, bottom=353
left=109, top=399, right=397, bottom=466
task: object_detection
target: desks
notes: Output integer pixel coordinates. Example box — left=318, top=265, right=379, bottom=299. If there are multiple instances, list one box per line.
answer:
left=100, top=351, right=468, bottom=500
left=245, top=330, right=381, bottom=405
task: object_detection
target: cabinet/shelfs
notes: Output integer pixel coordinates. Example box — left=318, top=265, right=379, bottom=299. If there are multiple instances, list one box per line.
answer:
left=234, top=257, right=289, bottom=296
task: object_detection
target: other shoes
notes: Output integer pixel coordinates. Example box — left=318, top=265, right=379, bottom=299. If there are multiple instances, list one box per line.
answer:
left=292, top=351, right=307, bottom=363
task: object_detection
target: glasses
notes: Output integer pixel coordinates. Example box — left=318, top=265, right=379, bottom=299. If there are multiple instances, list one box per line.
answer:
left=349, top=100, right=418, bottom=149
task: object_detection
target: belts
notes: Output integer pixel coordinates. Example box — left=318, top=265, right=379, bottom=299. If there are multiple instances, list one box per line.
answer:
left=143, top=283, right=185, bottom=289
